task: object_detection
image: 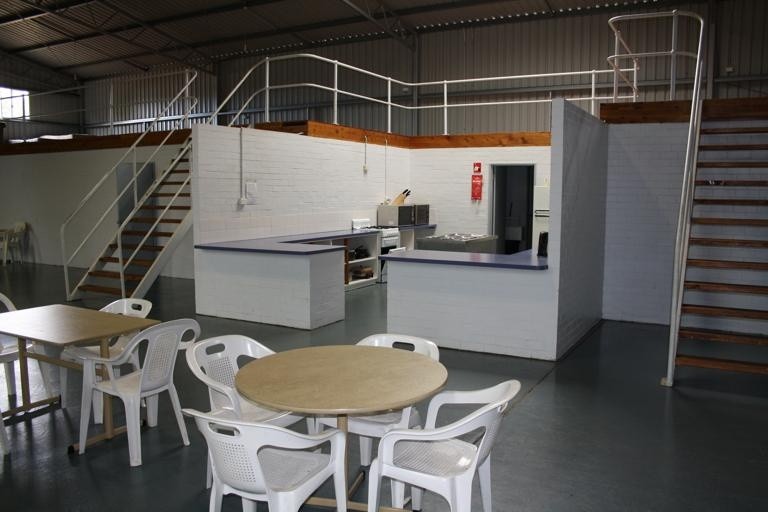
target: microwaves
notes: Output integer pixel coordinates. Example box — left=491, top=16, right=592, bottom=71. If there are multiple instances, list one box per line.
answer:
left=415, top=204, right=430, bottom=226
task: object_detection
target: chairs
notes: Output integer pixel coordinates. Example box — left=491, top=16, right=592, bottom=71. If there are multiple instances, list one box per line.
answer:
left=179, top=407, right=348, bottom=512
left=0, top=292, right=54, bottom=408
left=78, top=317, right=201, bottom=467
left=185, top=334, right=315, bottom=490
left=367, top=379, right=522, bottom=512
left=0, top=222, right=27, bottom=265
left=59, top=297, right=153, bottom=425
left=314, top=333, right=440, bottom=510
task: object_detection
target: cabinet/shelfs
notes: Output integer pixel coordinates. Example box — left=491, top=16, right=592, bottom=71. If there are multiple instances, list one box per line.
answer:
left=399, top=224, right=437, bottom=250
left=377, top=248, right=549, bottom=361
left=380, top=227, right=406, bottom=283
left=193, top=229, right=383, bottom=330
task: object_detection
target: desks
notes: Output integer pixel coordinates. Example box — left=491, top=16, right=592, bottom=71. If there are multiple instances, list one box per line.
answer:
left=234, top=344, right=449, bottom=512
left=416, top=232, right=499, bottom=255
left=0, top=303, right=162, bottom=454
